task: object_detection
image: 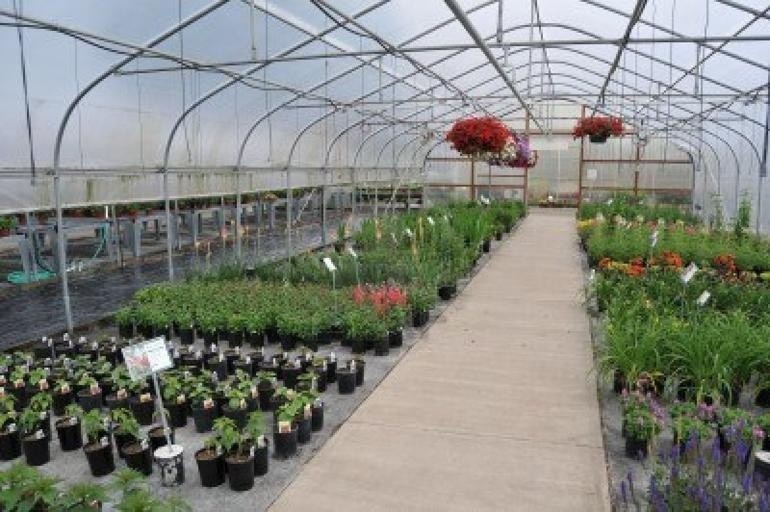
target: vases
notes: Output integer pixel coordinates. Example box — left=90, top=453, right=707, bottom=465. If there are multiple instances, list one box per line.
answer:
left=718, top=422, right=732, bottom=453
left=762, top=437, right=769, bottom=450
left=590, top=132, right=606, bottom=143
left=626, top=437, right=647, bottom=459
left=674, top=429, right=689, bottom=454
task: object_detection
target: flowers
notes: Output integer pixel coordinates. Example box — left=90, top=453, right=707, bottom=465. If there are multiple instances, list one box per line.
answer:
left=618, top=387, right=770, bottom=439
left=598, top=250, right=736, bottom=280
left=571, top=115, right=626, bottom=142
left=445, top=115, right=536, bottom=168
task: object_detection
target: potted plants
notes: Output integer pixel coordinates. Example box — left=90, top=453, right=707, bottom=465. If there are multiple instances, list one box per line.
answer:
left=584, top=294, right=769, bottom=408
left=580, top=226, right=692, bottom=268
